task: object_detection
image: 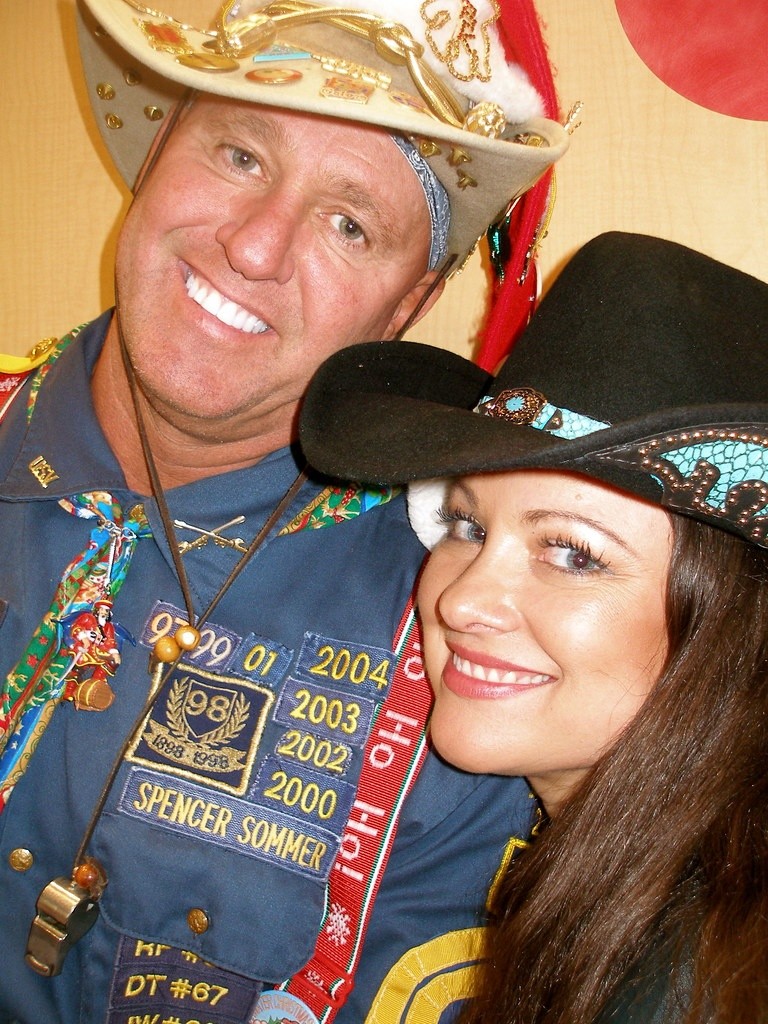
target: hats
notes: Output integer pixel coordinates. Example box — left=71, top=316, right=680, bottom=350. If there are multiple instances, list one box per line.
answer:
left=287, top=229, right=768, bottom=551
left=75, top=0, right=574, bottom=374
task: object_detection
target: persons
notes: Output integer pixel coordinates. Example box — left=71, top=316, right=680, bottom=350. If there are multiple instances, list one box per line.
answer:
left=290, top=231, right=768, bottom=1024
left=0, top=0, right=559, bottom=1024
